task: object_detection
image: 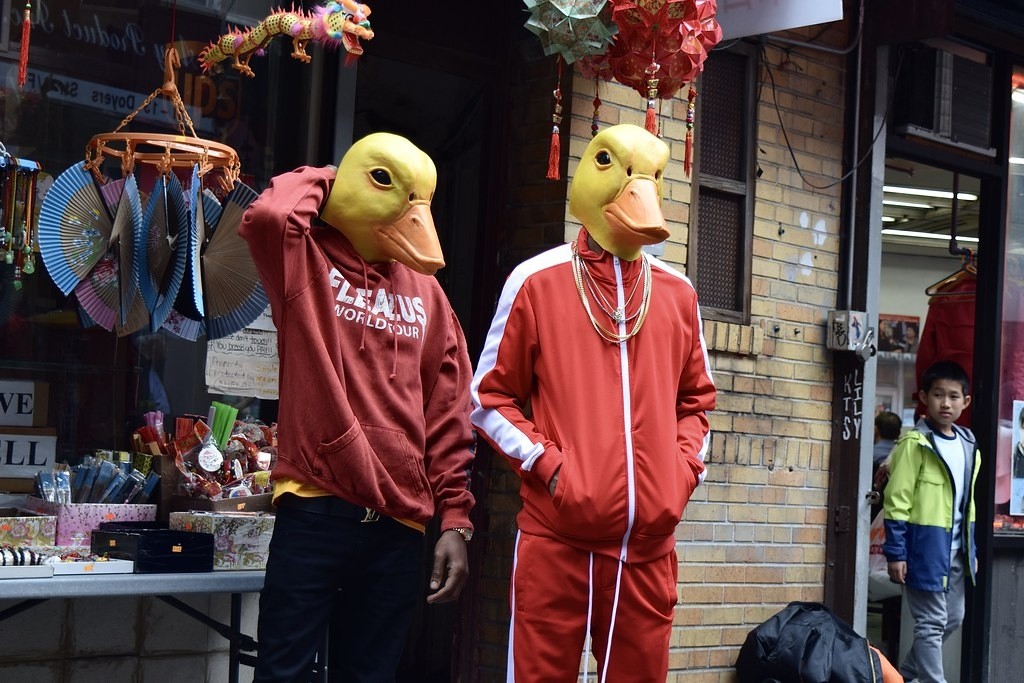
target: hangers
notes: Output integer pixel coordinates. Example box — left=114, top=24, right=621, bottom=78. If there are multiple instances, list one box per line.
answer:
left=925, top=248, right=977, bottom=297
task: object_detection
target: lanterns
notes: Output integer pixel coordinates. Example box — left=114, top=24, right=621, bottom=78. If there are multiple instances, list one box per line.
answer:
left=519, top=0, right=724, bottom=179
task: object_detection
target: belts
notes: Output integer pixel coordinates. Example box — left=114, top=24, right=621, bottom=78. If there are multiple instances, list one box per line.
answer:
left=287, top=494, right=382, bottom=523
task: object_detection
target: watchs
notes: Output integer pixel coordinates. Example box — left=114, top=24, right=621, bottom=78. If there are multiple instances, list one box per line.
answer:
left=452, top=528, right=473, bottom=541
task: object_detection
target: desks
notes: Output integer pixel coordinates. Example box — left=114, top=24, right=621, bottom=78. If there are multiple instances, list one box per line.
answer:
left=0, top=570, right=265, bottom=683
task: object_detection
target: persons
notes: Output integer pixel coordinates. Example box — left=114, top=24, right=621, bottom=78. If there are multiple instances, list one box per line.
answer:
left=470, top=123, right=717, bottom=683
left=874, top=411, right=903, bottom=462
left=884, top=360, right=981, bottom=683
left=239, top=131, right=476, bottom=683
left=877, top=320, right=919, bottom=354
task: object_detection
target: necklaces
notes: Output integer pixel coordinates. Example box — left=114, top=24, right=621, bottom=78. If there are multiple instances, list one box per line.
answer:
left=571, top=238, right=652, bottom=344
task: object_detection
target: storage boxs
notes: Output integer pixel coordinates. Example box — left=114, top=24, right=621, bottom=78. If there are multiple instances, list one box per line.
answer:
left=91, top=530, right=216, bottom=575
left=168, top=511, right=276, bottom=572
left=0, top=506, right=58, bottom=548
left=27, top=494, right=158, bottom=547
left=150, top=455, right=186, bottom=521
left=0, top=380, right=49, bottom=427
left=0, top=426, right=58, bottom=493
left=173, top=493, right=274, bottom=512
